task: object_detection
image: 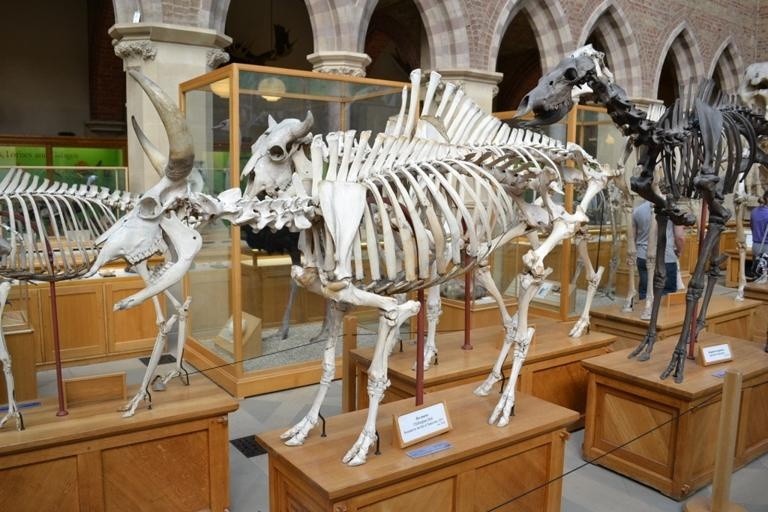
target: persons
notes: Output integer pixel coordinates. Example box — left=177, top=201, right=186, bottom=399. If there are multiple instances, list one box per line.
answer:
left=750, top=190, right=768, bottom=264
left=631, top=201, right=686, bottom=300
